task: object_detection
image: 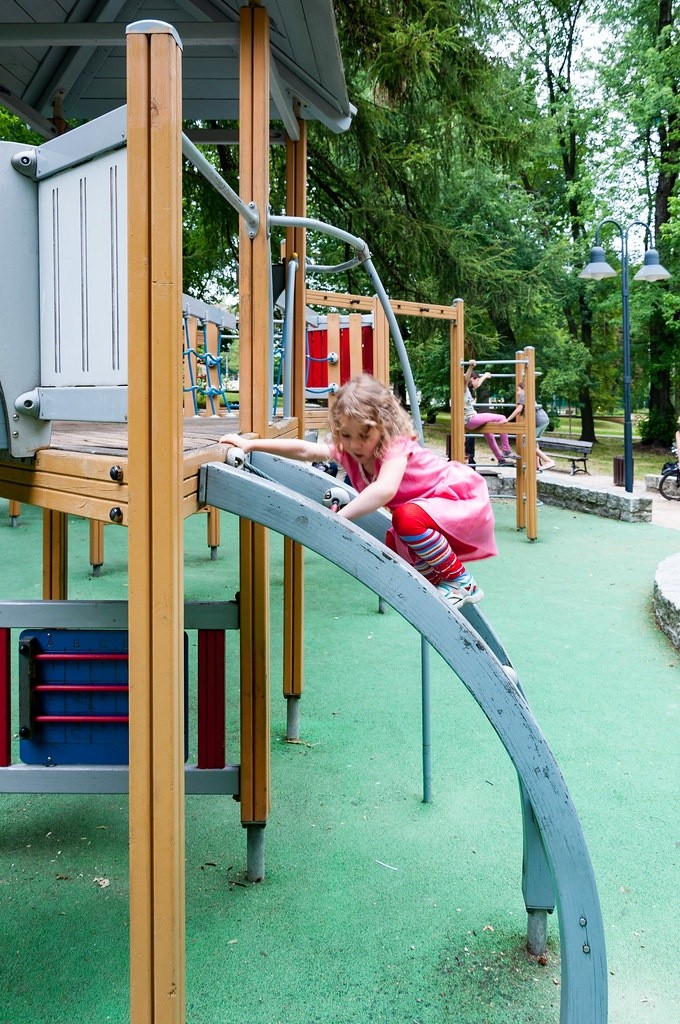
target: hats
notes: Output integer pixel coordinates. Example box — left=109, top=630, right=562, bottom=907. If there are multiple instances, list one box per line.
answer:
left=676, top=416, right=680, bottom=423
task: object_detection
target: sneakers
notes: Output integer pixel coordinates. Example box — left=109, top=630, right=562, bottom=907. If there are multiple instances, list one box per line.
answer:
left=436, top=572, right=484, bottom=609
left=498, top=460, right=515, bottom=466
left=502, top=450, right=521, bottom=460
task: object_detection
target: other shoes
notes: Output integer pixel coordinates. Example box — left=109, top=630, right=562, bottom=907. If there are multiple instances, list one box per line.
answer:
left=539, top=461, right=556, bottom=470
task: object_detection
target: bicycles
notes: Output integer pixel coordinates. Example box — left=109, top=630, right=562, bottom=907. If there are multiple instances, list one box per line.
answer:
left=659, top=441, right=680, bottom=501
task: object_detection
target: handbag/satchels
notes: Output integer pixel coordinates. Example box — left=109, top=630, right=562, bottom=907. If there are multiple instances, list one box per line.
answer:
left=661, top=462, right=679, bottom=476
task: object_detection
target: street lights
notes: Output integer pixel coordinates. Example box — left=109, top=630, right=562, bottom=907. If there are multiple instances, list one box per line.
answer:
left=578, top=219, right=673, bottom=491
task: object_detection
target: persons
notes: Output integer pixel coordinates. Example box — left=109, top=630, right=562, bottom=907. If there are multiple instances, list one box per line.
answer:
left=220, top=375, right=495, bottom=608
left=464, top=360, right=556, bottom=470
left=675, top=416, right=680, bottom=470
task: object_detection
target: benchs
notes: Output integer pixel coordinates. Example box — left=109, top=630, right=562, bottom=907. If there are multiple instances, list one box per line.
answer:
left=538, top=437, right=594, bottom=475
